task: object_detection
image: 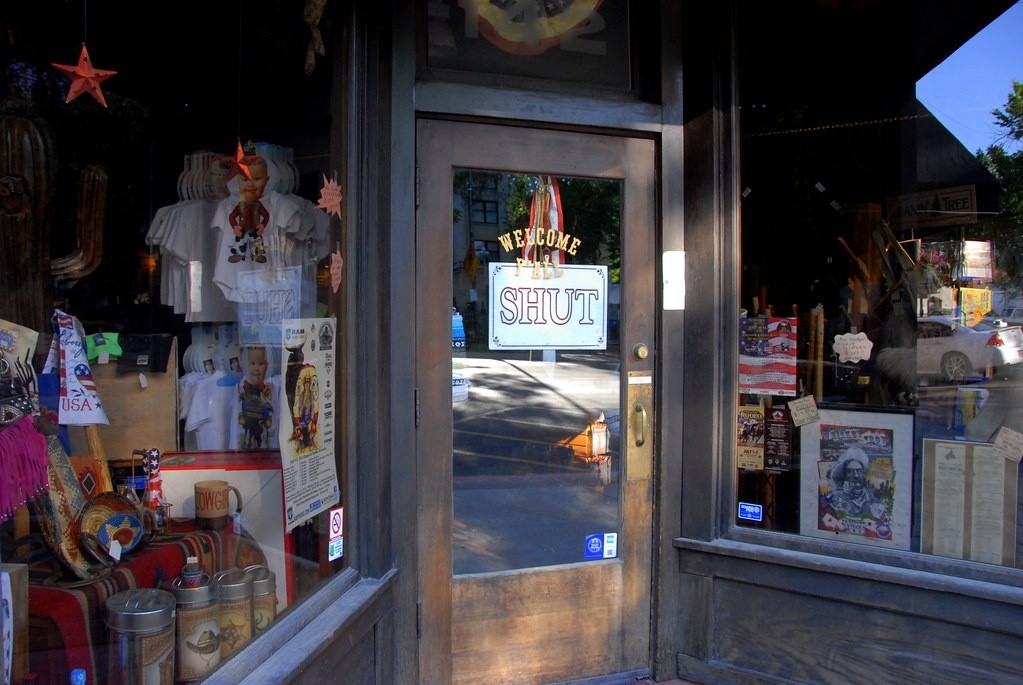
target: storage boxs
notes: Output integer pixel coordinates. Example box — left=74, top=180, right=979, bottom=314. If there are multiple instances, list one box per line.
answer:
left=159, top=448, right=297, bottom=615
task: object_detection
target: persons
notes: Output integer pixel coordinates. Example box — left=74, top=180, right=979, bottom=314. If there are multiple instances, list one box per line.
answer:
left=248, top=346, right=268, bottom=384
left=239, top=156, right=270, bottom=199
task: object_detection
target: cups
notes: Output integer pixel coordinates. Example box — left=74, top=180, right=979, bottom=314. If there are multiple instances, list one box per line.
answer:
left=152, top=503, right=173, bottom=536
left=194, top=480, right=243, bottom=530
left=131, top=501, right=158, bottom=547
left=104, top=566, right=277, bottom=685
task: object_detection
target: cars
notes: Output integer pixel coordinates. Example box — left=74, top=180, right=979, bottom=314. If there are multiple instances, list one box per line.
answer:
left=917, top=306, right=1023, bottom=383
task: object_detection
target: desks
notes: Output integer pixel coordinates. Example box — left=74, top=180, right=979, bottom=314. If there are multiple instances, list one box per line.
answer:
left=28, top=517, right=277, bottom=685
